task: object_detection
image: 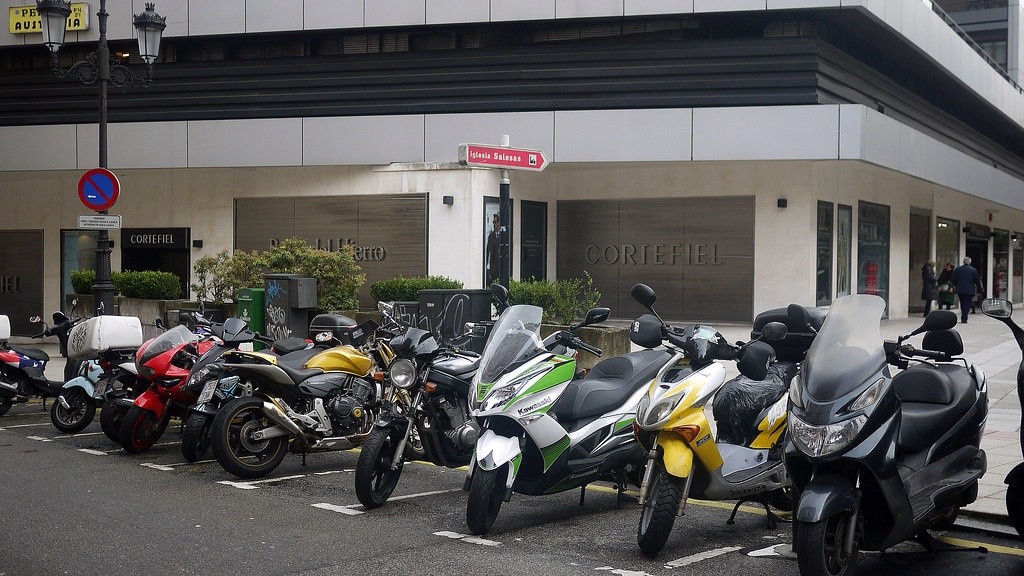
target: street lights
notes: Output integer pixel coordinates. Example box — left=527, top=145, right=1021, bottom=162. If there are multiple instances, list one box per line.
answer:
left=34, top=0, right=169, bottom=319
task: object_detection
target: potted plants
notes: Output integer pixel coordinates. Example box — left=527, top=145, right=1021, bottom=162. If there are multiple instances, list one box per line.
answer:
left=69, top=269, right=95, bottom=294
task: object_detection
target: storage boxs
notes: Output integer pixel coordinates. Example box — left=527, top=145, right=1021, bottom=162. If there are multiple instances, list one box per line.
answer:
left=0, top=314, right=10, bottom=339
left=309, top=314, right=358, bottom=346
left=67, top=315, right=142, bottom=357
left=750, top=306, right=828, bottom=363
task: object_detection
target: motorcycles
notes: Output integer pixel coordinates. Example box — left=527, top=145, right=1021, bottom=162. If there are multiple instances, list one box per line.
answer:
left=354, top=301, right=587, bottom=509
left=49, top=300, right=360, bottom=464
left=197, top=315, right=431, bottom=481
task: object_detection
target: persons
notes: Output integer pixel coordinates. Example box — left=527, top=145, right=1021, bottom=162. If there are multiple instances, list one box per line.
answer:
left=484, top=212, right=509, bottom=288
left=970, top=275, right=985, bottom=314
left=995, top=264, right=1004, bottom=298
left=838, top=223, right=847, bottom=292
left=817, top=224, right=830, bottom=300
left=921, top=260, right=939, bottom=319
left=937, top=262, right=957, bottom=311
left=951, top=256, right=982, bottom=324
left=864, top=260, right=878, bottom=294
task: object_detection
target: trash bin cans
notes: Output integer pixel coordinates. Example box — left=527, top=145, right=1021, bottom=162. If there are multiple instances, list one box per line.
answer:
left=236, top=286, right=266, bottom=351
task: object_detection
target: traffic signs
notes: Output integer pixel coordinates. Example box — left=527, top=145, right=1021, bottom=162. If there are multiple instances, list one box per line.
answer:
left=461, top=144, right=552, bottom=173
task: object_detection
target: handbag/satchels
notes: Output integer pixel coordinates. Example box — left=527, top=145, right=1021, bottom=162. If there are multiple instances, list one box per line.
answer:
left=940, top=284, right=949, bottom=293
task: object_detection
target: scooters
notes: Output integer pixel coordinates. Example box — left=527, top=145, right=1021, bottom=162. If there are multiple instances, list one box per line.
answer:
left=981, top=296, right=1024, bottom=539
left=462, top=282, right=683, bottom=536
left=631, top=282, right=831, bottom=559
left=785, top=294, right=990, bottom=576
left=0, top=298, right=84, bottom=418
